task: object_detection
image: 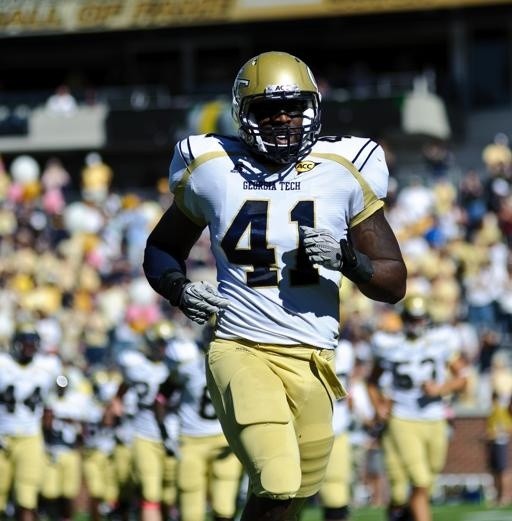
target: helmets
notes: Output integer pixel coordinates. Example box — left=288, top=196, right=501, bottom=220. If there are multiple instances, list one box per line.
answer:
left=11, top=323, right=40, bottom=354
left=145, top=322, right=175, bottom=348
left=232, top=52, right=323, bottom=165
left=401, top=296, right=432, bottom=330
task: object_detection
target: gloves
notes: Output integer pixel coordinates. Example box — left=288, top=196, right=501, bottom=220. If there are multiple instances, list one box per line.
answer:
left=178, top=281, right=228, bottom=324
left=300, top=226, right=344, bottom=270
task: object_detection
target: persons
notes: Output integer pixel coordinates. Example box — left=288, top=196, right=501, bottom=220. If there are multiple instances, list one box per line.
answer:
left=1, top=77, right=510, bottom=521
left=141, top=49, right=408, bottom=521
